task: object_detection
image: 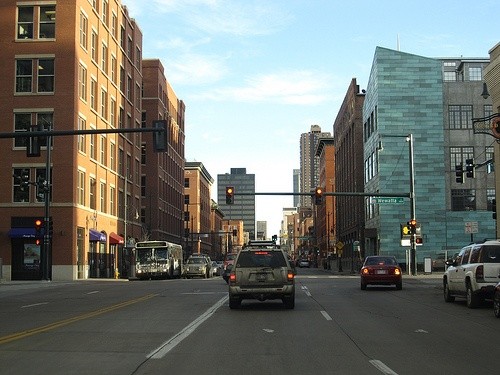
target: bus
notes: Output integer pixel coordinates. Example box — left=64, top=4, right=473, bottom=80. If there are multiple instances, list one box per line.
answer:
left=130, top=240, right=184, bottom=280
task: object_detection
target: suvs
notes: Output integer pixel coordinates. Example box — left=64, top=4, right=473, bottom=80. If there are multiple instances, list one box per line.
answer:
left=229, top=243, right=295, bottom=309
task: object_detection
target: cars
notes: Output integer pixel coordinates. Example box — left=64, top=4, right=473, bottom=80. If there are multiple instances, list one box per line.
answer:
left=299, top=259, right=310, bottom=267
left=361, top=255, right=402, bottom=291
left=182, top=252, right=236, bottom=279
left=493, top=273, right=500, bottom=319
left=442, top=241, right=500, bottom=308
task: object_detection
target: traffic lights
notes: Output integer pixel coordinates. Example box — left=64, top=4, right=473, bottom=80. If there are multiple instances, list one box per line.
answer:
left=38, top=177, right=45, bottom=194
left=20, top=169, right=28, bottom=187
left=35, top=219, right=41, bottom=226
left=274, top=235, right=277, bottom=240
left=315, top=188, right=323, bottom=205
left=272, top=236, right=275, bottom=241
left=36, top=239, right=40, bottom=245
left=407, top=220, right=416, bottom=234
left=455, top=162, right=463, bottom=183
left=416, top=238, right=422, bottom=244
left=492, top=199, right=496, bottom=219
left=226, top=187, right=234, bottom=205
left=466, top=159, right=474, bottom=178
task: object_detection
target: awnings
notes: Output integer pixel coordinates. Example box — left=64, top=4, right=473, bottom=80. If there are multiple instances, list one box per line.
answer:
left=89, top=229, right=105, bottom=241
left=8, top=228, right=38, bottom=238
left=109, top=233, right=125, bottom=244
